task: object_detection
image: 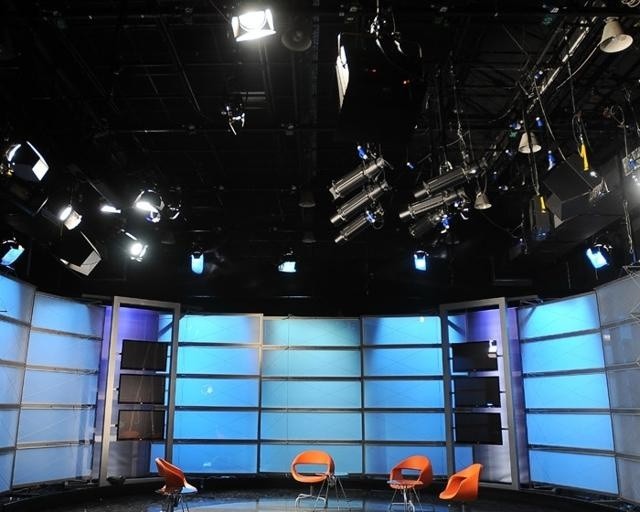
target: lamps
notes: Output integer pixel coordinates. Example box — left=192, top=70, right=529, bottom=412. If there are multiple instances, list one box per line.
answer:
left=596, top=15, right=634, bottom=54
left=100, top=278, right=100, bottom=281
left=519, top=131, right=542, bottom=156
left=0, top=138, right=206, bottom=283
left=413, top=250, right=431, bottom=271
left=276, top=254, right=301, bottom=274
left=229, top=1, right=276, bottom=43
left=473, top=192, right=492, bottom=212
left=280, top=16, right=315, bottom=53
left=586, top=235, right=614, bottom=272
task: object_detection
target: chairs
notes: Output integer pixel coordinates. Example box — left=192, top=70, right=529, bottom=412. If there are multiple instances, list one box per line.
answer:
left=291, top=450, right=335, bottom=507
left=438, top=463, right=484, bottom=512
left=155, top=455, right=197, bottom=512
left=390, top=455, right=433, bottom=510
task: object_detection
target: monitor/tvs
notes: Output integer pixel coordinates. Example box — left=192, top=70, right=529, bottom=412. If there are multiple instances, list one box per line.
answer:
left=118, top=374, right=165, bottom=405
left=454, top=377, right=501, bottom=408
left=455, top=412, right=502, bottom=445
left=120, top=339, right=168, bottom=371
left=117, top=410, right=165, bottom=441
left=453, top=340, right=498, bottom=372
